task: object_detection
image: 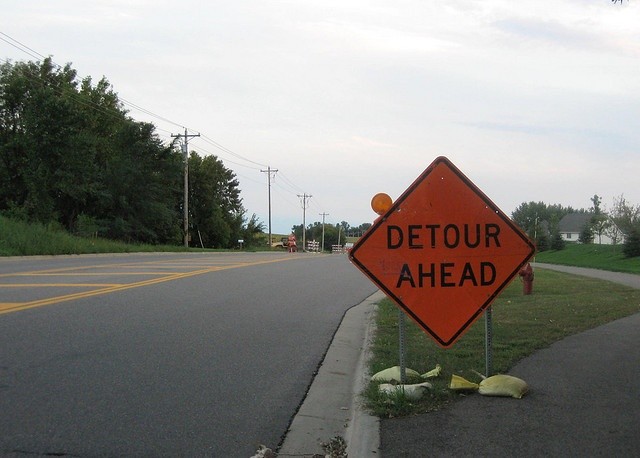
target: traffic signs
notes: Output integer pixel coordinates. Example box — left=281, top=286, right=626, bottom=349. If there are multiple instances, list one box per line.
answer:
left=349, top=156, right=535, bottom=349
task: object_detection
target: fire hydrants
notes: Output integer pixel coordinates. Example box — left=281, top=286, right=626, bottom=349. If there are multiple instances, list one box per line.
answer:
left=519, top=263, right=534, bottom=294
